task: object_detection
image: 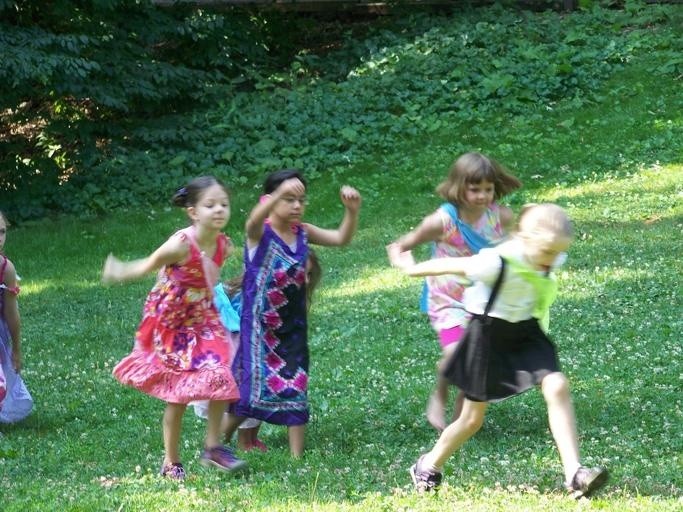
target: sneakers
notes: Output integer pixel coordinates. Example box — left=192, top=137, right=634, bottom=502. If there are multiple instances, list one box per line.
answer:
left=563, top=465, right=608, bottom=499
left=237, top=441, right=266, bottom=452
left=200, top=446, right=245, bottom=470
left=409, top=455, right=442, bottom=494
left=161, top=464, right=185, bottom=479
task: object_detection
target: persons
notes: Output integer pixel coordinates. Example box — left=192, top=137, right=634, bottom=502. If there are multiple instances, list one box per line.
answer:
left=103, top=175, right=248, bottom=483
left=395, top=204, right=609, bottom=501
left=213, top=168, right=361, bottom=458
left=386, top=152, right=521, bottom=432
left=1, top=213, right=34, bottom=425
